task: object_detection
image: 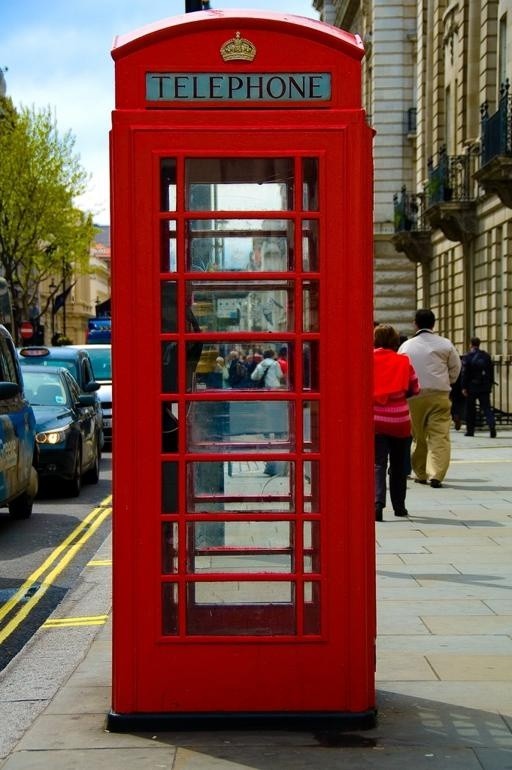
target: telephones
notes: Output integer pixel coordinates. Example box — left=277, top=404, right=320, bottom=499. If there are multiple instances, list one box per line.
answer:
left=161, top=286, right=203, bottom=403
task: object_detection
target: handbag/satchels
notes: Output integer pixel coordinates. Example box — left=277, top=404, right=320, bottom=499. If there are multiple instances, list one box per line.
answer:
left=257, top=379, right=265, bottom=388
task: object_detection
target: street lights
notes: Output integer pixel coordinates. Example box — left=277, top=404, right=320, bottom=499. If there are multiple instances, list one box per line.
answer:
left=49, top=278, right=57, bottom=337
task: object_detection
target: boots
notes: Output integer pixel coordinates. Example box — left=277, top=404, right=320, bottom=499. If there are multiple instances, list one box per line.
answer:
left=454, top=415, right=462, bottom=431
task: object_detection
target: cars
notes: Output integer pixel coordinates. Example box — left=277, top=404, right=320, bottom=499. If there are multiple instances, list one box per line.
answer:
left=0, top=317, right=112, bottom=519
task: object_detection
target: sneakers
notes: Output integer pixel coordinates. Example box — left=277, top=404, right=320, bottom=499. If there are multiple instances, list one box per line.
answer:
left=464, top=431, right=474, bottom=437
left=415, top=479, right=443, bottom=487
left=376, top=508, right=383, bottom=521
left=490, top=433, right=496, bottom=438
left=395, top=508, right=408, bottom=516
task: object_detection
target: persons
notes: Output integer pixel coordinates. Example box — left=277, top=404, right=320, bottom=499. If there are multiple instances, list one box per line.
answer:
left=397, top=308, right=463, bottom=488
left=374, top=324, right=420, bottom=523
left=460, top=336, right=497, bottom=438
left=449, top=354, right=466, bottom=430
left=216, top=344, right=310, bottom=477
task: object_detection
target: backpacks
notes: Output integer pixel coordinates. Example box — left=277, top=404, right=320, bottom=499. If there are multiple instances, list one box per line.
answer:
left=236, top=364, right=247, bottom=378
left=373, top=353, right=410, bottom=402
left=472, top=353, right=491, bottom=385
